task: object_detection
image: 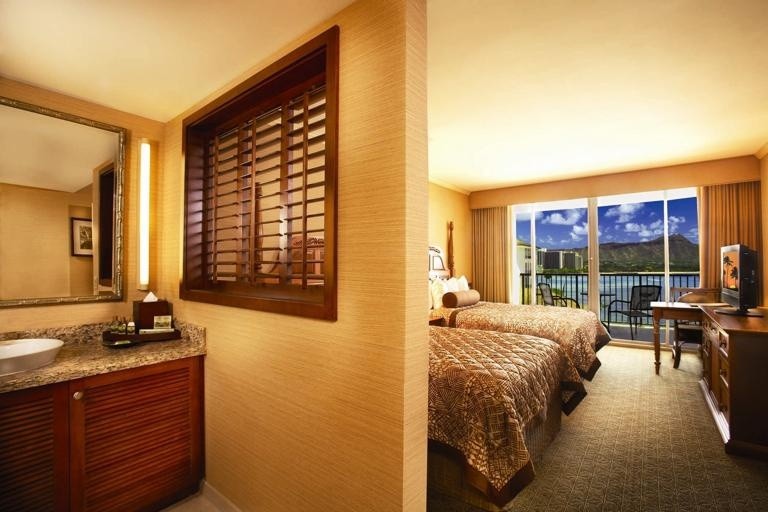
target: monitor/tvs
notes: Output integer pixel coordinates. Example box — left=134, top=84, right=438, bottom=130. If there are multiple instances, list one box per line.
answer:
left=713, top=244, right=764, bottom=317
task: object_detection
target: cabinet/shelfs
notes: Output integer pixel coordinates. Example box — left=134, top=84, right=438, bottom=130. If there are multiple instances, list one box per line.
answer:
left=698, top=305, right=768, bottom=456
left=1, top=355, right=205, bottom=511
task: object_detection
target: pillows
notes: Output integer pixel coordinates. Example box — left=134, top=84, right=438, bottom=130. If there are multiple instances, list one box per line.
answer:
left=442, top=289, right=480, bottom=308
left=431, top=277, right=446, bottom=309
left=447, top=276, right=458, bottom=293
left=458, top=275, right=468, bottom=290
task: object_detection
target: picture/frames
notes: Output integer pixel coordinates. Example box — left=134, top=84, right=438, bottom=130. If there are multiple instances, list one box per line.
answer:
left=70, top=216, right=94, bottom=257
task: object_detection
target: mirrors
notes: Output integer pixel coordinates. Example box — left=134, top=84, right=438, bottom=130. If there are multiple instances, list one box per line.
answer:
left=0, top=96, right=130, bottom=309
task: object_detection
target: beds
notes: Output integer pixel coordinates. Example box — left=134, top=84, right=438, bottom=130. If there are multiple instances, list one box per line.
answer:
left=428, top=220, right=612, bottom=382
left=254, top=234, right=588, bottom=510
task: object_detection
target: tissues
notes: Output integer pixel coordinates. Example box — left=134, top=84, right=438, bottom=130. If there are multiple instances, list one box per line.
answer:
left=133, top=292, right=176, bottom=329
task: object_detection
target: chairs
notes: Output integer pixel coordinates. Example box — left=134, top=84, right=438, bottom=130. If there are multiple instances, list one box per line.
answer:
left=670, top=286, right=721, bottom=369
left=607, top=285, right=662, bottom=340
left=538, top=283, right=580, bottom=308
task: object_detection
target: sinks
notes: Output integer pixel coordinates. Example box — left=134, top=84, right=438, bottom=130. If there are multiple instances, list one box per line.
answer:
left=0, top=335, right=64, bottom=376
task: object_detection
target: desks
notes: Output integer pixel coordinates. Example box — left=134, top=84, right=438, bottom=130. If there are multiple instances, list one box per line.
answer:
left=580, top=292, right=616, bottom=321
left=649, top=300, right=725, bottom=375
